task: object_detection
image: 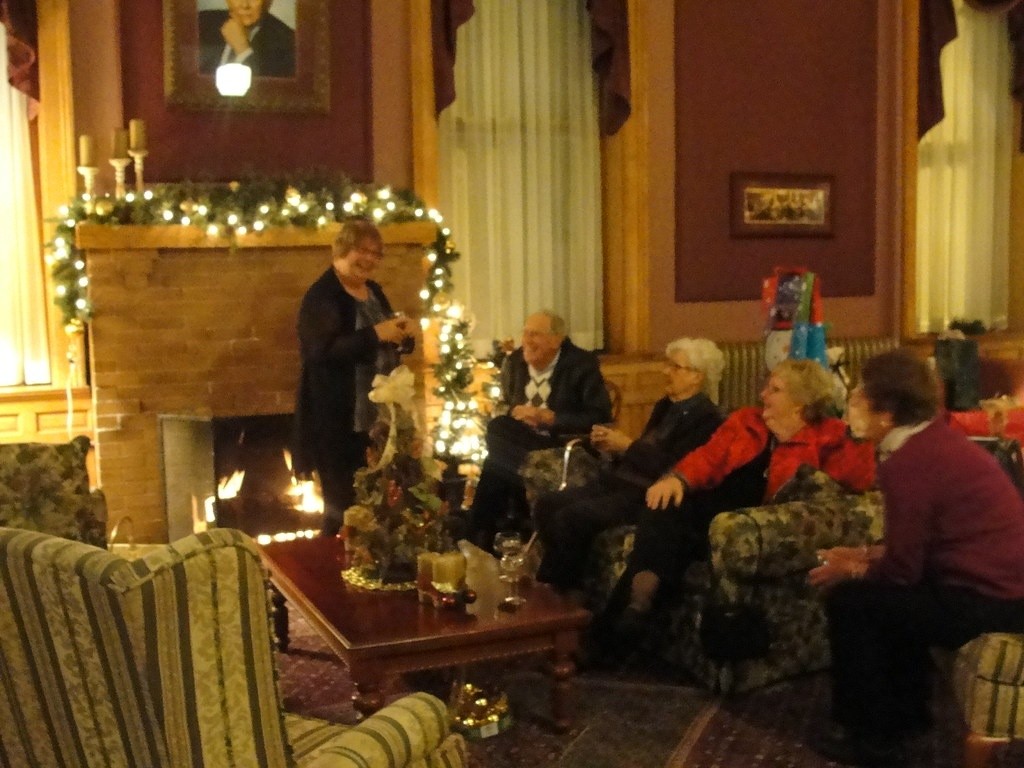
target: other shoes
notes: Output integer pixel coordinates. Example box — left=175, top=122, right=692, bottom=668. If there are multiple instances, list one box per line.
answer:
left=607, top=606, right=655, bottom=643
left=807, top=709, right=936, bottom=765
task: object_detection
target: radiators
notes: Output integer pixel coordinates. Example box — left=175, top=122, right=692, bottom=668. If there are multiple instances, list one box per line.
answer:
left=718, top=335, right=899, bottom=417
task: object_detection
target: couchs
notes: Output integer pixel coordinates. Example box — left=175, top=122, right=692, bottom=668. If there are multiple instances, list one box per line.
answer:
left=521, top=444, right=884, bottom=693
left=923, top=631, right=1024, bottom=768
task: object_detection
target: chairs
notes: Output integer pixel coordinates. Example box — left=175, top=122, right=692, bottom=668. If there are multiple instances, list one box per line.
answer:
left=0, top=434, right=471, bottom=768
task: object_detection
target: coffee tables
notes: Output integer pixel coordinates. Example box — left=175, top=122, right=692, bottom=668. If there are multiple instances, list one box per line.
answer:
left=263, top=535, right=595, bottom=738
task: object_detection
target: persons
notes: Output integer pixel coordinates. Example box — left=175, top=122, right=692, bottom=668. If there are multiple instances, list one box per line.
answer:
left=292, top=215, right=417, bottom=541
left=199, top=0, right=295, bottom=78
left=464, top=313, right=1024, bottom=768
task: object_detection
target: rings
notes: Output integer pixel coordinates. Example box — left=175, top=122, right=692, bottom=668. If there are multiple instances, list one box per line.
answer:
left=600, top=432, right=605, bottom=435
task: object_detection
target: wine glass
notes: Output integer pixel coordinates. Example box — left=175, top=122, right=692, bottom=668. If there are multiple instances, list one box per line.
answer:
left=500, top=553, right=529, bottom=605
left=494, top=531, right=521, bottom=582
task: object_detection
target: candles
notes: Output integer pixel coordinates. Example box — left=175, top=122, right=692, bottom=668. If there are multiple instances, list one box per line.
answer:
left=79, top=134, right=98, bottom=167
left=129, top=118, right=146, bottom=150
left=110, top=126, right=128, bottom=159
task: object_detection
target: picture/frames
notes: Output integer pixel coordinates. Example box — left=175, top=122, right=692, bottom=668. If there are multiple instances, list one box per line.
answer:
left=160, top=0, right=332, bottom=118
left=729, top=170, right=836, bottom=240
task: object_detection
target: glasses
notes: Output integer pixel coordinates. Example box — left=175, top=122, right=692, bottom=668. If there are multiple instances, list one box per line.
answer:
left=764, top=381, right=787, bottom=396
left=516, top=328, right=546, bottom=341
left=350, top=243, right=384, bottom=260
left=663, top=360, right=697, bottom=376
